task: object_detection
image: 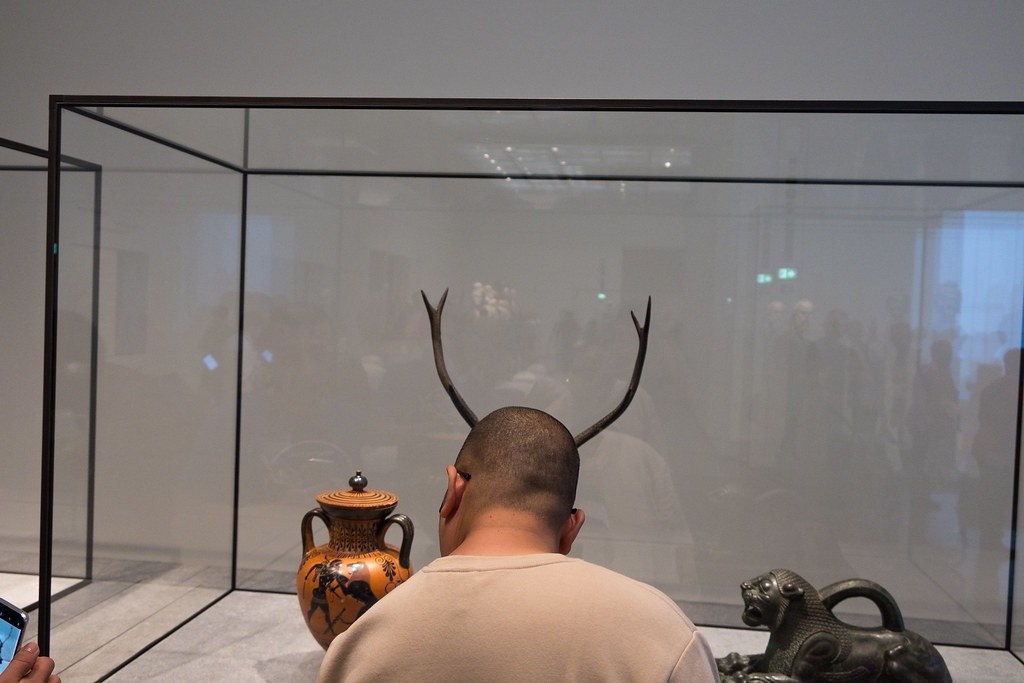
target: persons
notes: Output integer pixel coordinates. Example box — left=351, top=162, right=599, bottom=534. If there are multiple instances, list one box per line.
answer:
left=0, top=641, right=63, bottom=683
left=797, top=315, right=1022, bottom=563
left=552, top=411, right=700, bottom=599
left=315, top=407, right=721, bottom=683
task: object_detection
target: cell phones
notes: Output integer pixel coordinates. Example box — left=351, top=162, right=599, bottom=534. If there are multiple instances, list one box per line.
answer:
left=0, top=598, right=28, bottom=675
